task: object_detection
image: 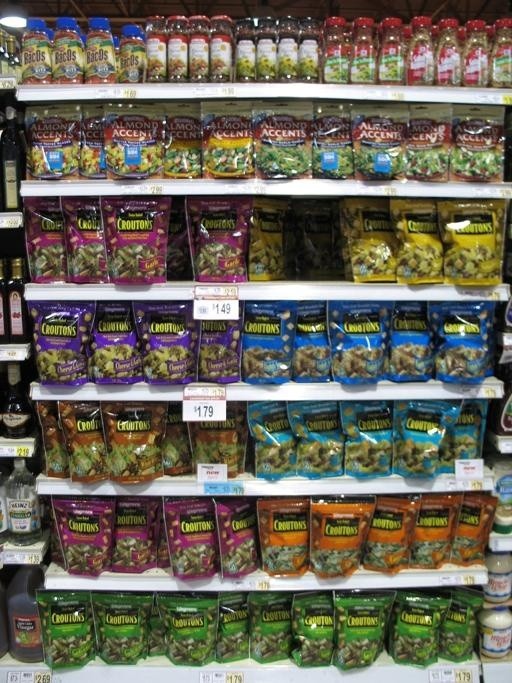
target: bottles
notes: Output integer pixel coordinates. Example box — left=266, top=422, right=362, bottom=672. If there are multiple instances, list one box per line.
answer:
left=3, top=564, right=56, bottom=664
left=0, top=15, right=512, bottom=91
left=1, top=101, right=37, bottom=549
